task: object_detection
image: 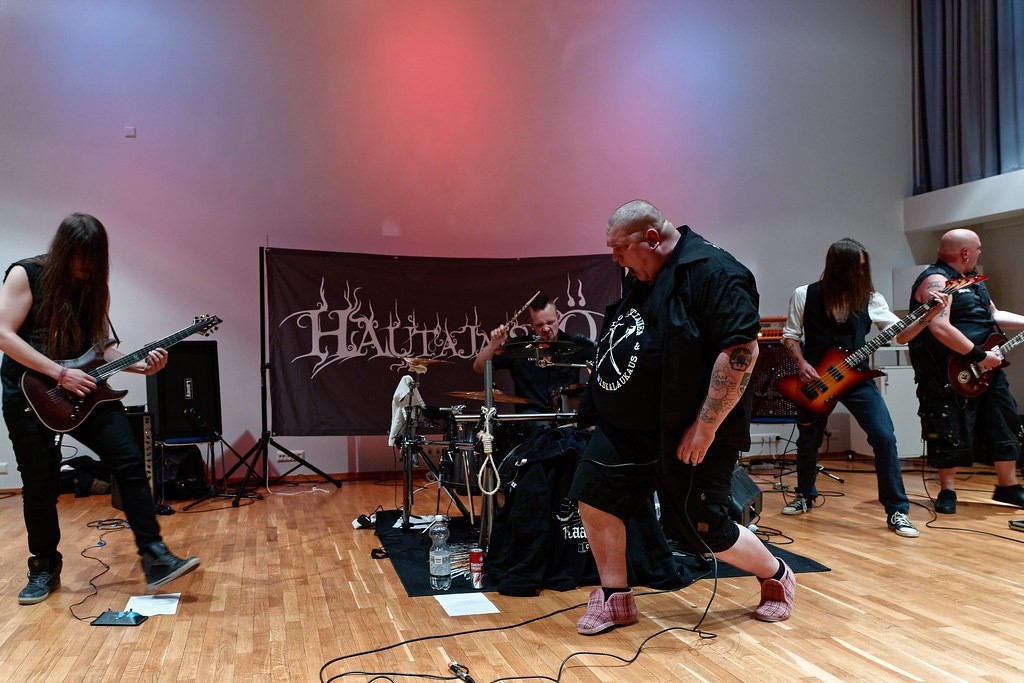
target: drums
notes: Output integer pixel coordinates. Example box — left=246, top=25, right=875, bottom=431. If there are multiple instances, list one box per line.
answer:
left=551, top=382, right=593, bottom=428
left=437, top=410, right=501, bottom=493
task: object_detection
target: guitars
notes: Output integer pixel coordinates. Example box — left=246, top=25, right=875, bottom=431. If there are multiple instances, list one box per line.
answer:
left=19, top=313, right=225, bottom=435
left=773, top=273, right=990, bottom=417
left=945, top=329, right=1024, bottom=400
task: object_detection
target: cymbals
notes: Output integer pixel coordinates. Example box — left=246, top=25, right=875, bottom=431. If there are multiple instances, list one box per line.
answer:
left=448, top=388, right=538, bottom=405
left=370, top=354, right=458, bottom=387
left=493, top=340, right=577, bottom=359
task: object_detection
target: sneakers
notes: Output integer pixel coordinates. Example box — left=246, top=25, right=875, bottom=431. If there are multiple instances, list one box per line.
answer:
left=992, top=484, right=1024, bottom=506
left=780, top=492, right=812, bottom=515
left=754, top=556, right=796, bottom=622
left=19, top=556, right=63, bottom=604
left=935, top=489, right=956, bottom=513
left=576, top=586, right=638, bottom=634
left=139, top=543, right=200, bottom=590
left=887, top=510, right=920, bottom=538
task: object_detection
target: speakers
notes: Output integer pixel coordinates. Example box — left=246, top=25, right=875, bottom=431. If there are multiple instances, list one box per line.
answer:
left=145, top=341, right=223, bottom=445
left=750, top=342, right=806, bottom=424
left=112, top=412, right=154, bottom=512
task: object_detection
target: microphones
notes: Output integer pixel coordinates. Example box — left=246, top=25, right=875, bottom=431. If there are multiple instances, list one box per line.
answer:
left=184, top=409, right=195, bottom=414
left=448, top=662, right=476, bottom=683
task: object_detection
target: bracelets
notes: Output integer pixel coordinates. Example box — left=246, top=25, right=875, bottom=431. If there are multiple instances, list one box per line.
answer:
left=55, top=366, right=68, bottom=389
left=963, top=346, right=987, bottom=365
left=920, top=314, right=932, bottom=325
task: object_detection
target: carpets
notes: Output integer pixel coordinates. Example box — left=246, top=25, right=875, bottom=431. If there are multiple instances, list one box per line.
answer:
left=374, top=508, right=831, bottom=599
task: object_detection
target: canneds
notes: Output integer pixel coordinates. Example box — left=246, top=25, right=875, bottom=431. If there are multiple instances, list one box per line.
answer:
left=469, top=548, right=485, bottom=590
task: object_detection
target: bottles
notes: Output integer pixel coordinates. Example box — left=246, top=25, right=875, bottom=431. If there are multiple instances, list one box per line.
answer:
left=428, top=515, right=452, bottom=590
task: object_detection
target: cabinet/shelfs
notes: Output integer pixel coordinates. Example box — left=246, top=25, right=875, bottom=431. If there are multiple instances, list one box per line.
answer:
left=845, top=264, right=984, bottom=463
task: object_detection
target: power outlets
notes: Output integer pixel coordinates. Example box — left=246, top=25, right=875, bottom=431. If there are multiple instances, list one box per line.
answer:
left=277, top=450, right=305, bottom=463
left=751, top=433, right=780, bottom=443
left=825, top=430, right=840, bottom=439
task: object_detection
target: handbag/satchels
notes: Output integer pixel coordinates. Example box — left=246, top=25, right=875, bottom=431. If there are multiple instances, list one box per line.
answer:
left=151, top=443, right=207, bottom=499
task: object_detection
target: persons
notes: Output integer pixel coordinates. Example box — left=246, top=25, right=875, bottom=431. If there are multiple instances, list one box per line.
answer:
left=473, top=293, right=599, bottom=414
left=0, top=211, right=200, bottom=604
left=908, top=229, right=1024, bottom=514
left=570, top=200, right=796, bottom=635
left=780, top=236, right=950, bottom=538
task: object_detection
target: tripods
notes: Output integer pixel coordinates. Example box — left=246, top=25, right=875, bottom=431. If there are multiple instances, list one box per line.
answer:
left=182, top=410, right=265, bottom=511
